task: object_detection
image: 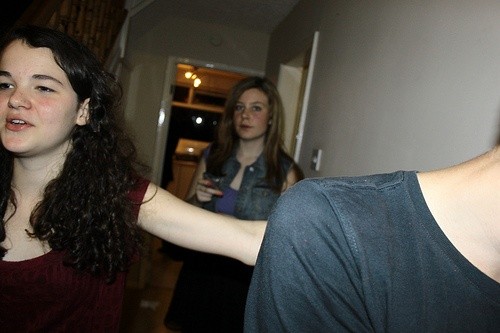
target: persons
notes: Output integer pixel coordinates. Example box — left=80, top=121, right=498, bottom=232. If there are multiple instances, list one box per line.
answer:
left=164, top=77, right=304, bottom=333
left=0, top=25, right=269, bottom=333
left=243, top=136, right=500, bottom=333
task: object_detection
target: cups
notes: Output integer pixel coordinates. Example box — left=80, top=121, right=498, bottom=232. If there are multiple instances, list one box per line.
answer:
left=203, top=172, right=231, bottom=194
